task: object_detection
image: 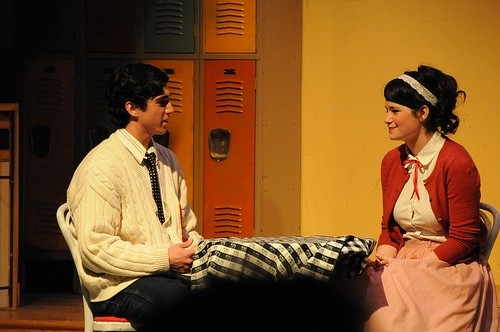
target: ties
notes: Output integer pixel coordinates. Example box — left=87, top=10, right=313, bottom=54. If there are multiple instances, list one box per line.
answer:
left=144, top=151, right=164, bottom=225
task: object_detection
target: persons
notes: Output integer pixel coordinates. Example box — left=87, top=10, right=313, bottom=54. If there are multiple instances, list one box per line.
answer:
left=66, top=62, right=203, bottom=332
left=358, top=64, right=500, bottom=332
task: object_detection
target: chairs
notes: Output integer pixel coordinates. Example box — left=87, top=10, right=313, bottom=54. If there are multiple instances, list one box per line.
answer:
left=56, top=203, right=138, bottom=332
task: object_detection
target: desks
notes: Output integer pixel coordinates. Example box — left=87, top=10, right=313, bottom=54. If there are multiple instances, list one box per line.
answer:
left=190, top=235, right=377, bottom=283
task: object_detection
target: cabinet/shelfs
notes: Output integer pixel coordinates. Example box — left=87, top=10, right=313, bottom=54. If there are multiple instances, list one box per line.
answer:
left=0, top=0, right=262, bottom=295
